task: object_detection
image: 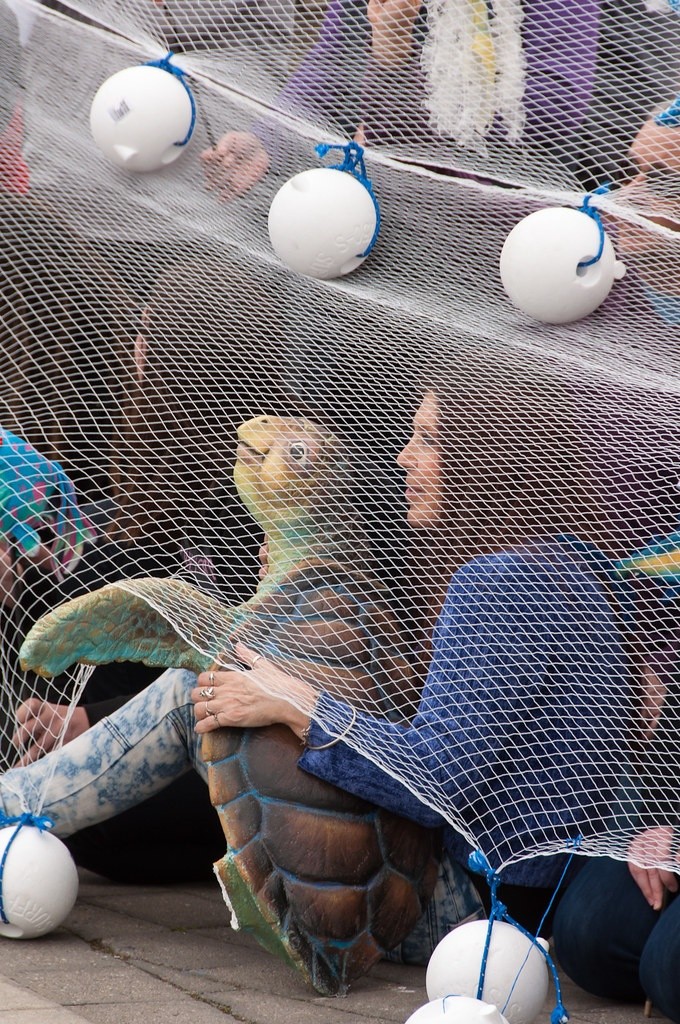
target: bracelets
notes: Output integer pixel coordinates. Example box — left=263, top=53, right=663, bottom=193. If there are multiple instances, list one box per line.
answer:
left=299, top=704, right=357, bottom=751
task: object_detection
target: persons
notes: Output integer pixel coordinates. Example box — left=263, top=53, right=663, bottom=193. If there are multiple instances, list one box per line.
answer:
left=0, top=0, right=680, bottom=1023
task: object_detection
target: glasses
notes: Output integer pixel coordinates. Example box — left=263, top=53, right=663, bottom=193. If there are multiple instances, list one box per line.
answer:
left=618, top=156, right=680, bottom=198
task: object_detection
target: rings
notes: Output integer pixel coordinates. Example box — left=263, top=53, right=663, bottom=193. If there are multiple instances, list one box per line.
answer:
left=199, top=687, right=215, bottom=700
left=214, top=710, right=221, bottom=728
left=205, top=702, right=213, bottom=715
left=252, top=655, right=263, bottom=666
left=210, top=671, right=214, bottom=685
